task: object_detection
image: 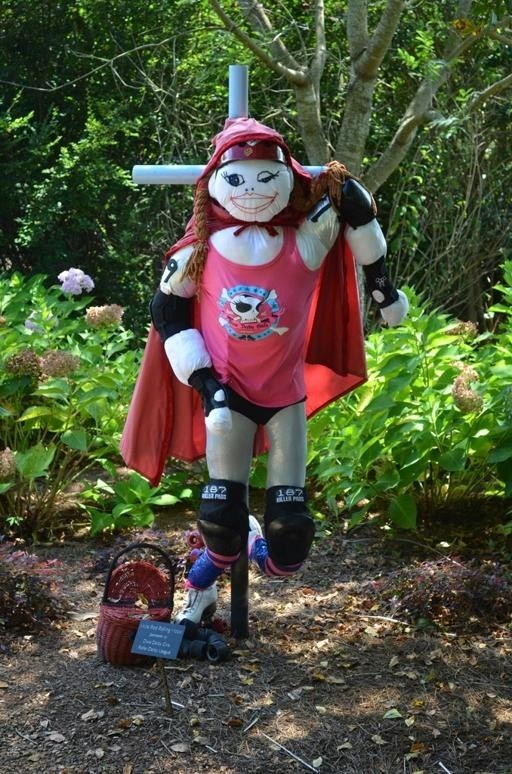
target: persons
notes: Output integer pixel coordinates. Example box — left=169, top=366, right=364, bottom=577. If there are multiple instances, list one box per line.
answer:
left=146, top=116, right=411, bottom=625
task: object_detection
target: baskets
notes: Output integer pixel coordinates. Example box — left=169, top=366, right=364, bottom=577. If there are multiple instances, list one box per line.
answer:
left=96, top=541, right=175, bottom=666
